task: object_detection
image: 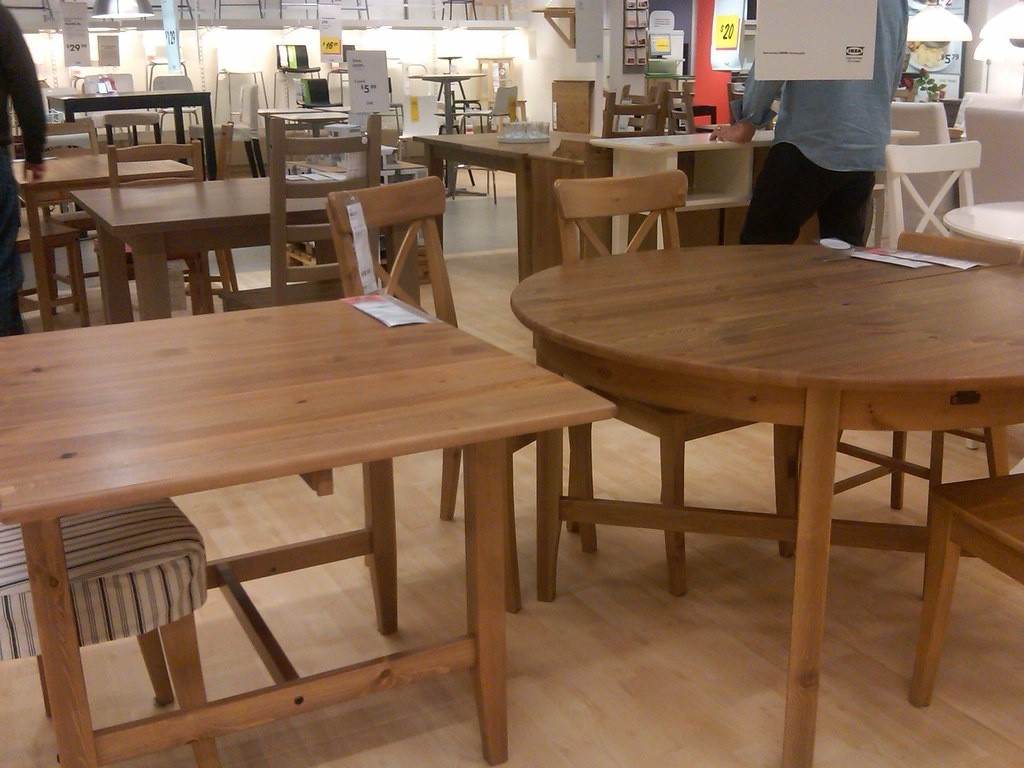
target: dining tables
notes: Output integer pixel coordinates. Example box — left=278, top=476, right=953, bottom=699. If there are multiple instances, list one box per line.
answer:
left=410, top=130, right=612, bottom=285
left=942, top=201, right=1024, bottom=245
left=508, top=243, right=1024, bottom=768
left=46, top=90, right=217, bottom=182
left=70, top=169, right=345, bottom=326
left=10, top=154, right=195, bottom=332
left=0, top=293, right=618, bottom=768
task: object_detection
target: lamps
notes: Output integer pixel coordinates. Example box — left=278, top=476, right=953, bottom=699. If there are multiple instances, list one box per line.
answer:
left=980, top=0, right=1024, bottom=49
left=905, top=0, right=974, bottom=43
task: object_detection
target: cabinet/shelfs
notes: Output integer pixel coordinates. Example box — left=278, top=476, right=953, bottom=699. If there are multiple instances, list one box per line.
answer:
left=590, top=128, right=921, bottom=254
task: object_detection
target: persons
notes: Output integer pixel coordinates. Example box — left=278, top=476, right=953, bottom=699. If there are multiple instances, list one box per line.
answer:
left=710, top=0, right=911, bottom=248
left=0, top=3, right=48, bottom=337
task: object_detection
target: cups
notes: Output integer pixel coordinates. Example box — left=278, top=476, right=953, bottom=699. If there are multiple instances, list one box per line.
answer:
left=498, top=121, right=549, bottom=140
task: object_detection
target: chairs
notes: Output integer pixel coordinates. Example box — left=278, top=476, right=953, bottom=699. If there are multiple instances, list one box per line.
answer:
left=963, top=106, right=1024, bottom=205
left=325, top=176, right=598, bottom=612
left=890, top=102, right=959, bottom=236
left=552, top=170, right=801, bottom=601
left=883, top=138, right=982, bottom=251
left=220, top=114, right=383, bottom=491
left=6, top=43, right=717, bottom=299
left=0, top=497, right=227, bottom=766
left=907, top=472, right=1023, bottom=710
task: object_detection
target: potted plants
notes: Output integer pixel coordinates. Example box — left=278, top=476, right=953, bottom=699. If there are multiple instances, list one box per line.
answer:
left=913, top=68, right=962, bottom=127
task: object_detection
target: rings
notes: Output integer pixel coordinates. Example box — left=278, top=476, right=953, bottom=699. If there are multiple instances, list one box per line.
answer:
left=718, top=126, right=720, bottom=130
